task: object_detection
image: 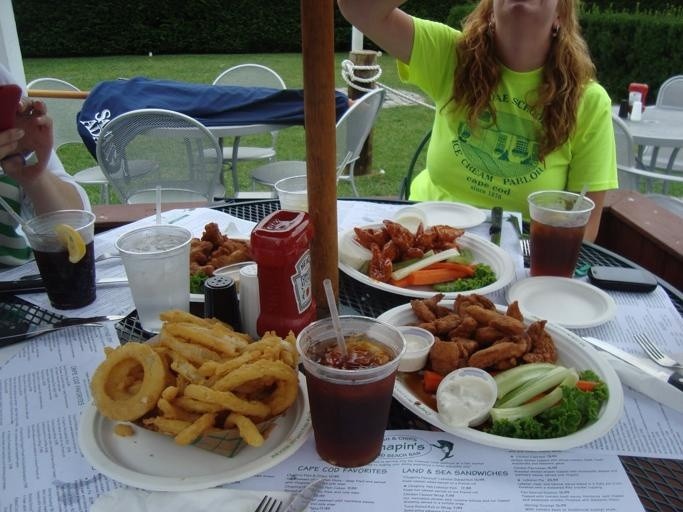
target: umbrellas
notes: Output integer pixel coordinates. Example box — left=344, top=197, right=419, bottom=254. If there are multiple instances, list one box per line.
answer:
left=24, top=74, right=373, bottom=175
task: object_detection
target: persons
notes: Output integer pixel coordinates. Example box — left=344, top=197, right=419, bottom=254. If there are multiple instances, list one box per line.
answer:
left=336, top=2, right=622, bottom=245
left=0, top=80, right=94, bottom=275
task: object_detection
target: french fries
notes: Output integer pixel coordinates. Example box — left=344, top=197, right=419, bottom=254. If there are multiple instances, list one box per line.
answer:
left=92, top=310, right=299, bottom=447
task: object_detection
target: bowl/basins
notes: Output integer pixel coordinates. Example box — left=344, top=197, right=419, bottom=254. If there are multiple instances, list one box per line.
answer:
left=212, top=258, right=256, bottom=293
left=392, top=207, right=429, bottom=232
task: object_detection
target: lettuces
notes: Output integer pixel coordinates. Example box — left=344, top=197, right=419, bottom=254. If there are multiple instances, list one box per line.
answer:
left=486, top=361, right=610, bottom=440
left=436, top=247, right=498, bottom=291
left=188, top=274, right=208, bottom=294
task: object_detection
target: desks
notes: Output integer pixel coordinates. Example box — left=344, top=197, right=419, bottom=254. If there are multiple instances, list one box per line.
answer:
left=613, top=107, right=683, bottom=194
left=151, top=122, right=291, bottom=191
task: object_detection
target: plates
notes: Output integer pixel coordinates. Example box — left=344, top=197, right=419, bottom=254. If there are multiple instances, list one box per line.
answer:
left=76, top=370, right=315, bottom=492
left=394, top=325, right=438, bottom=373
left=337, top=222, right=515, bottom=297
left=364, top=297, right=623, bottom=452
left=527, top=190, right=596, bottom=280
left=408, top=200, right=489, bottom=230
left=507, top=275, right=618, bottom=330
left=435, top=368, right=499, bottom=427
left=190, top=235, right=250, bottom=303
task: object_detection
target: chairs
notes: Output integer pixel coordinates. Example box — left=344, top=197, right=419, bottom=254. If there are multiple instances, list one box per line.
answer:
left=198, top=64, right=286, bottom=191
left=400, top=130, right=431, bottom=200
left=275, top=150, right=351, bottom=194
left=95, top=108, right=222, bottom=203
left=612, top=113, right=634, bottom=190
left=635, top=75, right=683, bottom=172
left=25, top=77, right=159, bottom=205
left=248, top=88, right=386, bottom=196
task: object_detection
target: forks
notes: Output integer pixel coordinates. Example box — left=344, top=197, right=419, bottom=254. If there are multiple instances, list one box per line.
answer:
left=633, top=331, right=683, bottom=370
left=254, top=495, right=283, bottom=512
left=508, top=215, right=531, bottom=256
left=20, top=251, right=120, bottom=280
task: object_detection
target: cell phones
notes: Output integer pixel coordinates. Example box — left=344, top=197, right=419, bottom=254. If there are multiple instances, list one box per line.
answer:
left=588, top=266, right=657, bottom=292
left=0, top=84, right=22, bottom=134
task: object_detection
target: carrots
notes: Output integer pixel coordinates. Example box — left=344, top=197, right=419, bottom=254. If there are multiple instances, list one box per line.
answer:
left=393, top=262, right=474, bottom=286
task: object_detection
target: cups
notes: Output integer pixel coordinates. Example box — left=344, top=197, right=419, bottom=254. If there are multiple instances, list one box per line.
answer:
left=23, top=209, right=97, bottom=309
left=274, top=175, right=308, bottom=216
left=114, top=223, right=194, bottom=336
left=295, top=312, right=406, bottom=469
left=629, top=92, right=642, bottom=113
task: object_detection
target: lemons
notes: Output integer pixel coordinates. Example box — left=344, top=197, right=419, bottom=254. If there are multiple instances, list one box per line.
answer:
left=53, top=224, right=88, bottom=264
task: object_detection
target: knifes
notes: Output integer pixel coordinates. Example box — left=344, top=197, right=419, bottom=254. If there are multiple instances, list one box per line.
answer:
left=0, top=277, right=129, bottom=292
left=489, top=206, right=504, bottom=248
left=283, top=478, right=325, bottom=512
left=0, top=315, right=127, bottom=340
left=583, top=334, right=683, bottom=392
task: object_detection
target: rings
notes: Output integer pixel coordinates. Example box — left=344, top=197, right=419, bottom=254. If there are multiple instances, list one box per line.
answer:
left=21, top=151, right=38, bottom=168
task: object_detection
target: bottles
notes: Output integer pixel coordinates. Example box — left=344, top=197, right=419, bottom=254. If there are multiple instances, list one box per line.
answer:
left=202, top=274, right=243, bottom=334
left=629, top=101, right=642, bottom=121
left=618, top=100, right=628, bottom=118
left=238, top=263, right=266, bottom=341
